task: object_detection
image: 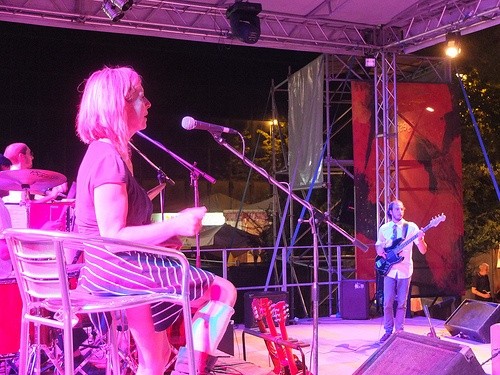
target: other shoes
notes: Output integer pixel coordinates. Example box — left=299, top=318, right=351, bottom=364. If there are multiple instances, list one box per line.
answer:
left=380, top=332, right=392, bottom=342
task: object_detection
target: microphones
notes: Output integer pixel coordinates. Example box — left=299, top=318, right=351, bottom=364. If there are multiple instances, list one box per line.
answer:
left=181, top=116, right=238, bottom=134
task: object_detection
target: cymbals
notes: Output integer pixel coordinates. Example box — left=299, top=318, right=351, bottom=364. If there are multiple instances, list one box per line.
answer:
left=0, top=167, right=69, bottom=192
left=52, top=199, right=75, bottom=209
left=147, top=183, right=167, bottom=199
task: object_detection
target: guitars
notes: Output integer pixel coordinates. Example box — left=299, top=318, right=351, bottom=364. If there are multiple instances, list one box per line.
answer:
left=252, top=297, right=313, bottom=375
left=374, top=211, right=447, bottom=277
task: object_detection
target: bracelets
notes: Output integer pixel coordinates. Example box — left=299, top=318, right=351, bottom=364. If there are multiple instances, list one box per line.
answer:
left=418, top=236, right=424, bottom=241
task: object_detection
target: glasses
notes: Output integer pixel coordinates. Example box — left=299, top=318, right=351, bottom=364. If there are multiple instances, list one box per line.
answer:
left=26, top=152, right=34, bottom=156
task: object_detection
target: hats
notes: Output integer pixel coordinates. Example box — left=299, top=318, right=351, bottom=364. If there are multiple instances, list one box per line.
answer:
left=4, top=143, right=28, bottom=162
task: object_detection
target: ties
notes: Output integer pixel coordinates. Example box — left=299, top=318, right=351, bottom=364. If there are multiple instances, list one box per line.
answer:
left=392, top=224, right=397, bottom=241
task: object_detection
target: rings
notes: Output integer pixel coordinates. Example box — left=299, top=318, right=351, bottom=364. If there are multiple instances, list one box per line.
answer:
left=382, top=252, right=384, bottom=254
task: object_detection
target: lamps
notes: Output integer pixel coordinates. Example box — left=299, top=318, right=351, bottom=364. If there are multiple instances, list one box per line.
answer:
left=444, top=30, right=461, bottom=57
left=101, top=0, right=124, bottom=21
left=111, top=0, right=133, bottom=11
left=364, top=55, right=376, bottom=67
left=225, top=0, right=263, bottom=44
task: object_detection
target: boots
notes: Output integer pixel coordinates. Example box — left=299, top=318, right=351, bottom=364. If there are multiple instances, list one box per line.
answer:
left=170, top=346, right=217, bottom=375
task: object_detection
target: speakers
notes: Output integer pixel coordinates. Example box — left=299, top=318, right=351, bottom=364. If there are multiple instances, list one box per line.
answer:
left=355, top=329, right=485, bottom=375
left=490, top=323, right=500, bottom=375
left=444, top=299, right=499, bottom=342
left=244, top=292, right=290, bottom=328
left=216, top=321, right=234, bottom=355
left=340, top=280, right=370, bottom=319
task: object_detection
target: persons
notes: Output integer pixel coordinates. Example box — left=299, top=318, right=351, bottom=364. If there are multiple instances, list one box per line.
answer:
left=375, top=200, right=427, bottom=342
left=0, top=154, right=113, bottom=375
left=55, top=67, right=237, bottom=375
left=3, top=143, right=60, bottom=203
left=471, top=263, right=493, bottom=302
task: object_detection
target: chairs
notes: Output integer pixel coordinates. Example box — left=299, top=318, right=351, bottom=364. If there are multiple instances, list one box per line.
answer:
left=1, top=228, right=196, bottom=375
